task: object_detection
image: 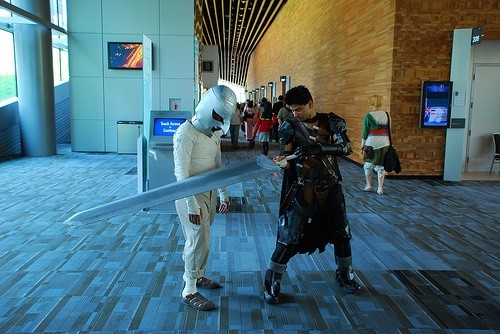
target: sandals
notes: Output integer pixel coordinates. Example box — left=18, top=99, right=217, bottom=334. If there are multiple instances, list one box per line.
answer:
left=182, top=291, right=215, bottom=311
left=197, top=276, right=220, bottom=289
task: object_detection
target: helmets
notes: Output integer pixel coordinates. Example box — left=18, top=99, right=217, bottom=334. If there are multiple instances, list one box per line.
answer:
left=196, top=84, right=237, bottom=134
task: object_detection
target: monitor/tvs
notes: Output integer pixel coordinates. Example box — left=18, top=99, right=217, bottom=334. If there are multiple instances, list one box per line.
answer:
left=108, top=42, right=143, bottom=70
left=419, top=81, right=453, bottom=129
left=149, top=110, right=192, bottom=150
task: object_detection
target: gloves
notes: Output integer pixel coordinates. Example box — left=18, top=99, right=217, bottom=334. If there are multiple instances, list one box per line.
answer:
left=294, top=143, right=321, bottom=158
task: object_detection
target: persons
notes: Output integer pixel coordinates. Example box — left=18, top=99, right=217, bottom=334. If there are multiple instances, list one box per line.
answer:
left=263, top=86, right=361, bottom=304
left=237, top=97, right=267, bottom=148
left=272, top=95, right=285, bottom=141
left=360, top=95, right=392, bottom=195
left=276, top=103, right=292, bottom=156
left=173, top=85, right=237, bottom=311
left=253, top=101, right=276, bottom=156
left=230, top=103, right=242, bottom=150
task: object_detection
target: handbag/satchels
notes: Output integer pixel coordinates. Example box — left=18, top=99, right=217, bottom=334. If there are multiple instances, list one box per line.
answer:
left=362, top=144, right=374, bottom=160
left=384, top=145, right=401, bottom=174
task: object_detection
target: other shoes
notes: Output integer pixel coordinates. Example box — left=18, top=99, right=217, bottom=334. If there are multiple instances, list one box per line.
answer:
left=376, top=190, right=383, bottom=194
left=361, top=187, right=372, bottom=192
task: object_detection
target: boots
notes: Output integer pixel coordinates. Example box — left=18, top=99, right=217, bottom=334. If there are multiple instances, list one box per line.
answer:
left=261, top=261, right=287, bottom=304
left=335, top=255, right=361, bottom=293
